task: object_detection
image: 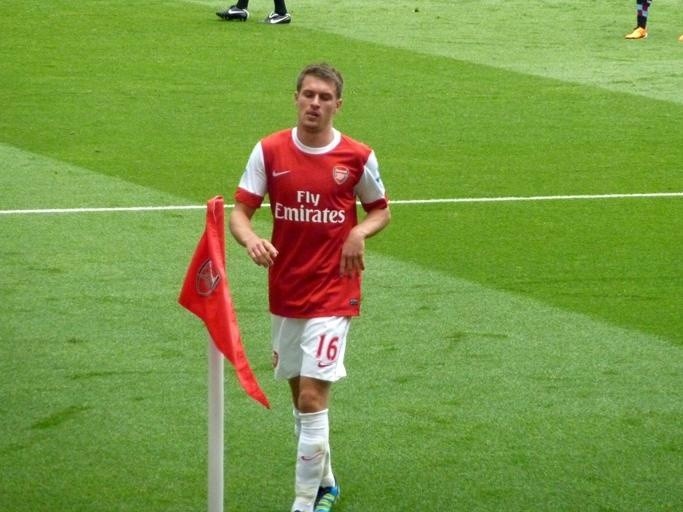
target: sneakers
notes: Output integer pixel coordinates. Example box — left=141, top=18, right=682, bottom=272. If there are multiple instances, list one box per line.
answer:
left=625, top=27, right=648, bottom=39
left=215, top=5, right=248, bottom=21
left=257, top=11, right=290, bottom=23
left=313, top=477, right=340, bottom=512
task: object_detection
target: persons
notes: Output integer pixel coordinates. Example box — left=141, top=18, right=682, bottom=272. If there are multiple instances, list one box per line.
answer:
left=230, top=62, right=392, bottom=512
left=215, top=0, right=292, bottom=25
left=624, top=0, right=683, bottom=42
